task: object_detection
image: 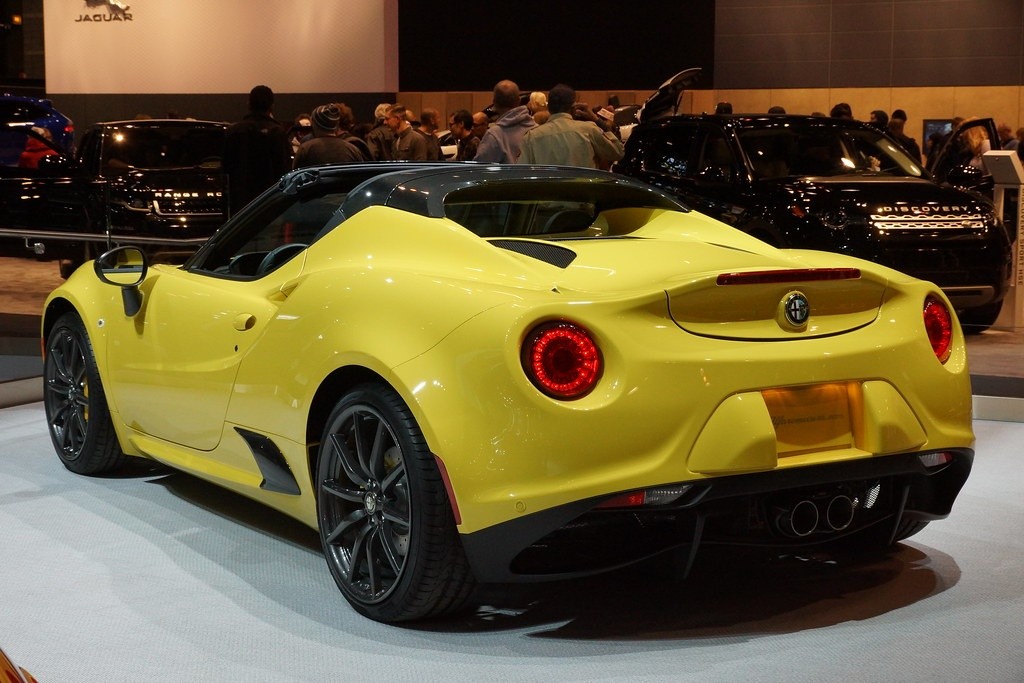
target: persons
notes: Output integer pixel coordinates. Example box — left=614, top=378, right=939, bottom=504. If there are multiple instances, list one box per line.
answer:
left=525, top=92, right=550, bottom=125
left=768, top=103, right=1024, bottom=180
left=473, top=80, right=539, bottom=165
left=449, top=109, right=488, bottom=161
left=290, top=103, right=446, bottom=245
left=220, top=85, right=290, bottom=208
left=715, top=102, right=732, bottom=114
left=516, top=85, right=624, bottom=234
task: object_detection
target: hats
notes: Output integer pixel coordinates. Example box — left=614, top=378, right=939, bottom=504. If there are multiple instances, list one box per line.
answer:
left=295, top=117, right=311, bottom=131
left=311, top=103, right=340, bottom=131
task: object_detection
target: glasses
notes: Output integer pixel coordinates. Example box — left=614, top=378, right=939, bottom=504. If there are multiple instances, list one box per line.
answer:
left=471, top=122, right=485, bottom=130
left=449, top=120, right=460, bottom=127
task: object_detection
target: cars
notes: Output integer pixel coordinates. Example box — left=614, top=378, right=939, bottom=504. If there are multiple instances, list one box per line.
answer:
left=40, top=162, right=974, bottom=630
left=79, top=117, right=235, bottom=268
left=609, top=67, right=1017, bottom=336
left=0, top=93, right=75, bottom=255
